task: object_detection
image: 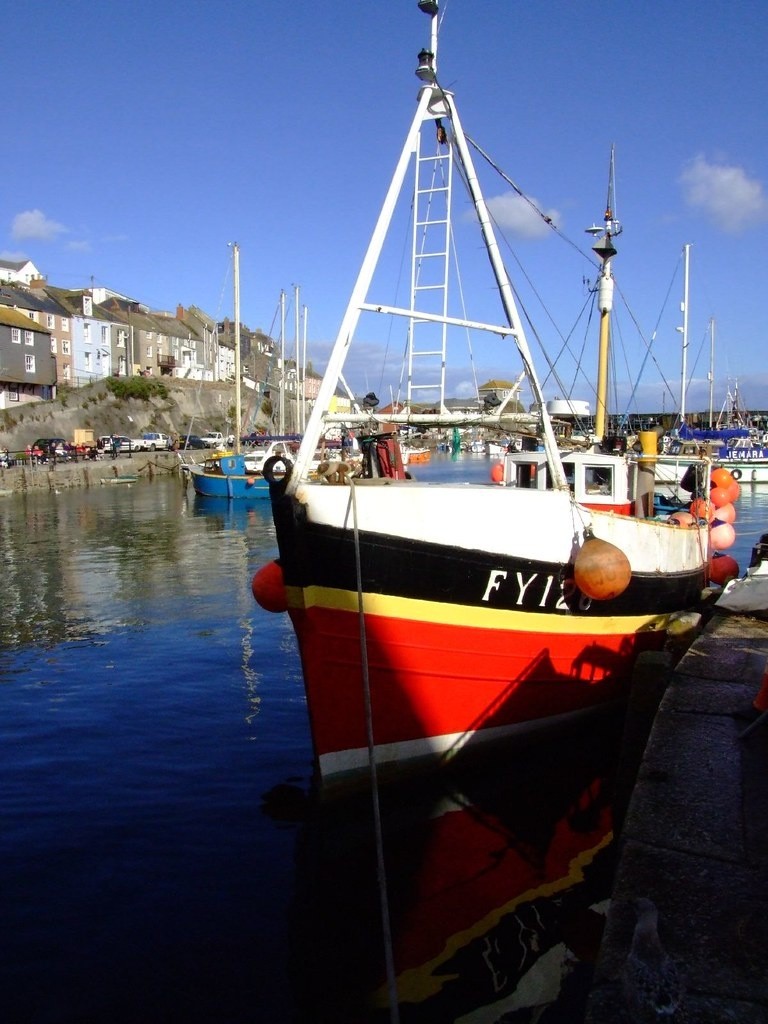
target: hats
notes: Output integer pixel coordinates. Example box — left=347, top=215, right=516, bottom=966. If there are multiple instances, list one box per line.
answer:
left=363, top=393, right=379, bottom=406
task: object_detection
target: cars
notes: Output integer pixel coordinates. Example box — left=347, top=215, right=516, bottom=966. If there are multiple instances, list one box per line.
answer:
left=179, top=435, right=205, bottom=450
left=99, top=435, right=141, bottom=452
left=31, top=438, right=74, bottom=453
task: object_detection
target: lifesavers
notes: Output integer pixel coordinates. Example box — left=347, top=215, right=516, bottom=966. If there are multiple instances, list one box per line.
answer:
left=700, top=586, right=723, bottom=628
left=616, top=650, right=673, bottom=806
left=731, top=469, right=742, bottom=480
left=664, top=610, right=704, bottom=669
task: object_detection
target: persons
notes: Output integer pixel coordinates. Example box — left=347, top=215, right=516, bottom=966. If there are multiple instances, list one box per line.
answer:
left=0, top=434, right=121, bottom=465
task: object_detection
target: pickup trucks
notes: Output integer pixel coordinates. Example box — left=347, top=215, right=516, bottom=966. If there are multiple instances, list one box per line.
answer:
left=132, top=433, right=171, bottom=452
left=200, top=432, right=229, bottom=449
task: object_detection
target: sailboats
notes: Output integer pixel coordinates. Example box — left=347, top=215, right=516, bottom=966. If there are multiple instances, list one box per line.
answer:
left=613, top=239, right=768, bottom=485
left=181, top=241, right=429, bottom=499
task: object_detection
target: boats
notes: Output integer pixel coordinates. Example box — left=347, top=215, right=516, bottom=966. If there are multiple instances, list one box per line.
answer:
left=100, top=475, right=139, bottom=483
left=258, top=0, right=737, bottom=802
left=714, top=534, right=768, bottom=621
